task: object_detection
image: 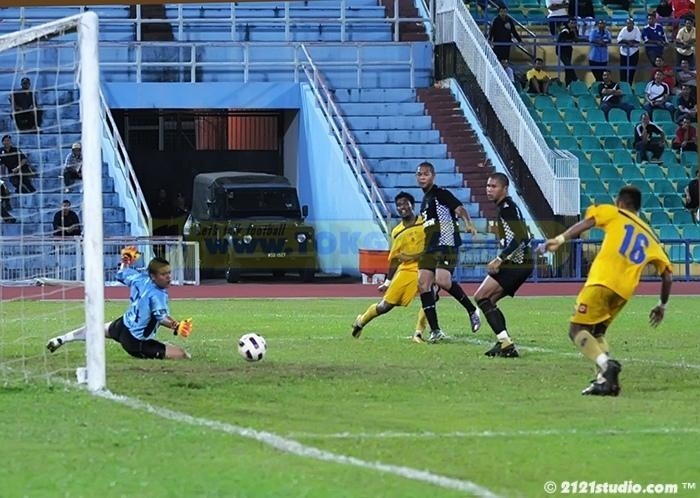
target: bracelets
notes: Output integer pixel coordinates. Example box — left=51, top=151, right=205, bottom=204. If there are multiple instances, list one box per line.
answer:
left=383, top=279, right=392, bottom=288
left=496, top=255, right=503, bottom=263
left=658, top=299, right=668, bottom=311
left=556, top=231, right=566, bottom=250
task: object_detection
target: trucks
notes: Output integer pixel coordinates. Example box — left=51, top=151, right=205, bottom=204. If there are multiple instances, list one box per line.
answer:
left=180, top=167, right=323, bottom=285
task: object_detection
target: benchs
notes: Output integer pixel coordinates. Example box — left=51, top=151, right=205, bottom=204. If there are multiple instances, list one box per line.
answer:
left=0, top=0, right=441, bottom=87
left=0, top=80, right=150, bottom=276
left=319, top=62, right=562, bottom=276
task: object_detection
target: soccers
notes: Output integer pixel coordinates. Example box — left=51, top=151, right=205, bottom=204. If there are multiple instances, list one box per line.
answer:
left=237, top=333, right=266, bottom=362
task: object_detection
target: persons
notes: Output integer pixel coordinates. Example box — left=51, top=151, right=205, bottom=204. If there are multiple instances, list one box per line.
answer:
left=10, top=77, right=43, bottom=131
left=351, top=190, right=431, bottom=344
left=683, top=170, right=699, bottom=208
left=42, top=244, right=195, bottom=360
left=471, top=171, right=536, bottom=359
left=0, top=134, right=35, bottom=192
left=62, top=142, right=84, bottom=187
left=53, top=200, right=83, bottom=235
left=539, top=186, right=675, bottom=399
left=417, top=162, right=483, bottom=342
left=0, top=163, right=17, bottom=221
left=487, top=0, right=699, bottom=162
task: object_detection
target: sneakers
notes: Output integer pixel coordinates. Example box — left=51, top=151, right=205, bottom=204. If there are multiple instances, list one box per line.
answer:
left=426, top=330, right=445, bottom=342
left=412, top=334, right=425, bottom=343
left=581, top=381, right=620, bottom=396
left=45, top=336, right=61, bottom=353
left=484, top=342, right=518, bottom=357
left=352, top=314, right=362, bottom=338
left=469, top=307, right=481, bottom=332
left=602, top=360, right=621, bottom=393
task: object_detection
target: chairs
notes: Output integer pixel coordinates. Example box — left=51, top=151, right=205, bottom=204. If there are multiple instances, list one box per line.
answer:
left=466, top=0, right=698, bottom=266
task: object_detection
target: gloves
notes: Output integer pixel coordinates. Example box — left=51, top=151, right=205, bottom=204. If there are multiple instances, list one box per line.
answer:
left=120, top=246, right=140, bottom=264
left=173, top=317, right=192, bottom=338
